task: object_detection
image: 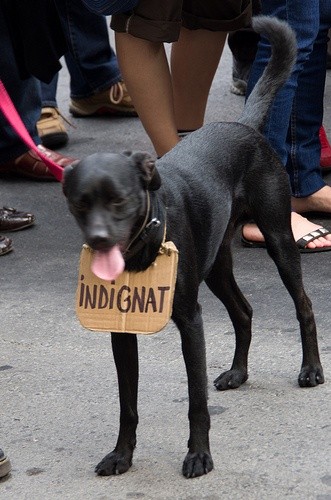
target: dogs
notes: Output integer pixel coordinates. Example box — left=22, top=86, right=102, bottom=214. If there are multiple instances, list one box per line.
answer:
left=61, top=15, right=325, bottom=479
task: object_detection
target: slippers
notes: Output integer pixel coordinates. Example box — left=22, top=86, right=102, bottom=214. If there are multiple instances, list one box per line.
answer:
left=241, top=227, right=330, bottom=253
left=301, top=211, right=331, bottom=221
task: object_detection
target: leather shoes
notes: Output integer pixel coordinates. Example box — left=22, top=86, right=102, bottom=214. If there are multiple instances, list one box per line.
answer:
left=0, top=234, right=13, bottom=255
left=0, top=144, right=80, bottom=180
left=0, top=206, right=34, bottom=232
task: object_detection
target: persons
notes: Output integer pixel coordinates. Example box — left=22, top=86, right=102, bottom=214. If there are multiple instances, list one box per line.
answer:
left=0, top=0, right=331, bottom=255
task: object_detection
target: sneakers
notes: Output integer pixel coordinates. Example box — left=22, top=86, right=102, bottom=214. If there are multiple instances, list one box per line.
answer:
left=69, top=78, right=136, bottom=117
left=36, top=107, right=69, bottom=148
